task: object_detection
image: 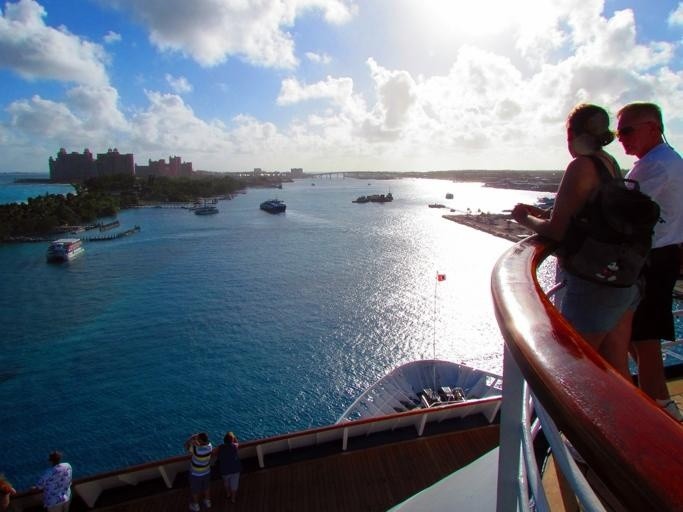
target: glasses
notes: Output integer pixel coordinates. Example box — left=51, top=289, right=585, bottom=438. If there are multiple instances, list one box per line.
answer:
left=616, top=121, right=656, bottom=135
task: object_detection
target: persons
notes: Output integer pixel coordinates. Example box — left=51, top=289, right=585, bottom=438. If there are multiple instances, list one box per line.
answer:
left=32, top=450, right=73, bottom=511
left=510, top=102, right=643, bottom=466
left=182, top=432, right=212, bottom=512
left=0, top=474, right=17, bottom=511
left=614, top=101, right=682, bottom=424
left=211, top=430, right=240, bottom=504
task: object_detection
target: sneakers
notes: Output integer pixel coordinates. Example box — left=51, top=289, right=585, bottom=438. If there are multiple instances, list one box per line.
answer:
left=189, top=504, right=200, bottom=511
left=204, top=501, right=212, bottom=508
left=662, top=400, right=683, bottom=422
left=226, top=493, right=236, bottom=503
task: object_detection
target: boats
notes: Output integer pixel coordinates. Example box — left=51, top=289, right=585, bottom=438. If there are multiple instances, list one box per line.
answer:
left=258, top=199, right=287, bottom=216
left=47, top=237, right=84, bottom=261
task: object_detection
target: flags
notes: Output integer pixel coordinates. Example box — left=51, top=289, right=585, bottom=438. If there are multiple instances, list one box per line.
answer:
left=436, top=274, right=446, bottom=282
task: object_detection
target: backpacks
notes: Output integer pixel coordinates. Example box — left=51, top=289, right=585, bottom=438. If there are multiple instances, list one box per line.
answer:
left=555, top=153, right=661, bottom=289
